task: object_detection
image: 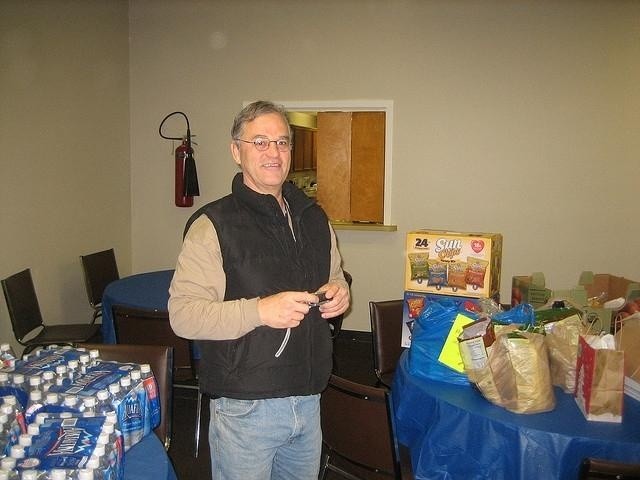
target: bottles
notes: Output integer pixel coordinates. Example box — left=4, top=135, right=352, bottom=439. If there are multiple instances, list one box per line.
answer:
left=0, top=397, right=125, bottom=480
left=27, top=361, right=160, bottom=452
left=0, top=342, right=100, bottom=396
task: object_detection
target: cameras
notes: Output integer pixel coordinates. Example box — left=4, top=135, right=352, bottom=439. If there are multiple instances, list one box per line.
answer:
left=307, top=292, right=331, bottom=309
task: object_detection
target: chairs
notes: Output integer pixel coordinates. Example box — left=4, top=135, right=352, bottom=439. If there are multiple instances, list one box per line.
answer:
left=80, top=248, right=120, bottom=324
left=320, top=298, right=412, bottom=479
left=1, top=267, right=104, bottom=359
left=173, top=336, right=205, bottom=458
left=77, top=344, right=177, bottom=452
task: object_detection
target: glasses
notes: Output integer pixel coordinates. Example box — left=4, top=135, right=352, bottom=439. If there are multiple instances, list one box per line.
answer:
left=236, top=137, right=292, bottom=152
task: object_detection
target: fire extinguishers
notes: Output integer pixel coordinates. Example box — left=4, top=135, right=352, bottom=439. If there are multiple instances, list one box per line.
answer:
left=158, top=112, right=197, bottom=207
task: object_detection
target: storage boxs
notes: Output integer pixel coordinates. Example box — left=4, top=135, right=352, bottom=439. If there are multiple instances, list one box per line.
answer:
left=401, top=229, right=503, bottom=350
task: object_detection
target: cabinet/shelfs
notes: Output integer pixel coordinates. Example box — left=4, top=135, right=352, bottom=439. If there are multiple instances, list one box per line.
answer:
left=289, top=123, right=317, bottom=172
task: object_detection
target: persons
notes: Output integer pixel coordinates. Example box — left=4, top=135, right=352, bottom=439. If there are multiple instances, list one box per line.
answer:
left=167, top=99, right=353, bottom=480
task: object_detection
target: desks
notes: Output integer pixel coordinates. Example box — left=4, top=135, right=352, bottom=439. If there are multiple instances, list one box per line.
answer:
left=123, top=430, right=170, bottom=459
left=388, top=347, right=640, bottom=480
left=104, top=268, right=204, bottom=429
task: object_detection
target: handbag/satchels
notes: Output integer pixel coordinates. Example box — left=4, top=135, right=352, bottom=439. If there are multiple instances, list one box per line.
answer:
left=572, top=314, right=626, bottom=424
